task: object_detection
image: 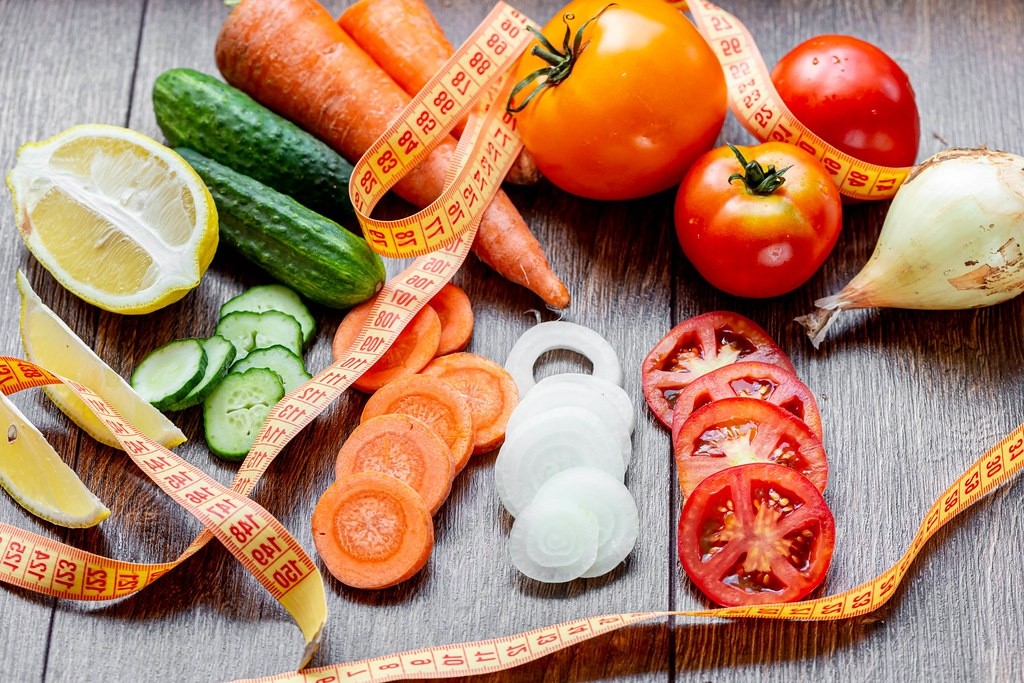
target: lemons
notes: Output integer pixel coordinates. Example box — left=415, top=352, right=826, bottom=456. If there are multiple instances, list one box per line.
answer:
left=1, top=123, right=219, bottom=529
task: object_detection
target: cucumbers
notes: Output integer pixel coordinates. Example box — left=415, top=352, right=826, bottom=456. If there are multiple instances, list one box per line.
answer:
left=127, top=69, right=385, bottom=456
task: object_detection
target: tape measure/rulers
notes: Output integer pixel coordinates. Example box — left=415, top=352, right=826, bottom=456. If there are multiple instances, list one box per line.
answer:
left=0, top=1, right=1023, bottom=683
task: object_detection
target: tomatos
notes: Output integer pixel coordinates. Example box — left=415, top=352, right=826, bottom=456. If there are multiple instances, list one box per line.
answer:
left=512, top=0, right=920, bottom=299
left=641, top=311, right=834, bottom=603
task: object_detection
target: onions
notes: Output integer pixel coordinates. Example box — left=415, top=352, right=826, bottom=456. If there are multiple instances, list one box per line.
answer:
left=804, top=151, right=1024, bottom=350
left=502, top=321, right=637, bottom=583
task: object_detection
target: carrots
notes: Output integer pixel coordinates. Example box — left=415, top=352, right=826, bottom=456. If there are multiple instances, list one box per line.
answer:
left=315, top=284, right=515, bottom=589
left=206, top=1, right=567, bottom=304
